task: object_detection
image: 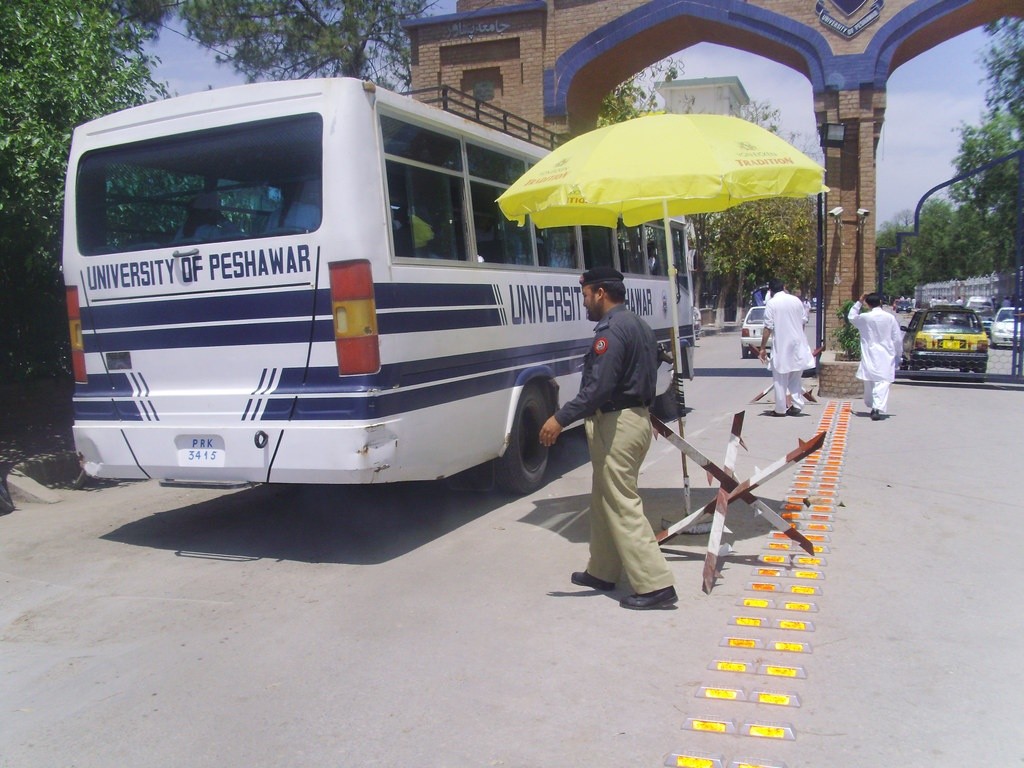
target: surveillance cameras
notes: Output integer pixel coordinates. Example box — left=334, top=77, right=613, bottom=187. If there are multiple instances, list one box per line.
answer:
left=828, top=207, right=844, bottom=217
left=857, top=208, right=870, bottom=217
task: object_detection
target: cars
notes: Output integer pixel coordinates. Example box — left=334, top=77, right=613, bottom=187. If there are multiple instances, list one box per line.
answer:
left=811, top=301, right=817, bottom=311
left=893, top=299, right=911, bottom=313
left=740, top=305, right=774, bottom=359
left=899, top=304, right=990, bottom=384
left=989, top=306, right=1022, bottom=350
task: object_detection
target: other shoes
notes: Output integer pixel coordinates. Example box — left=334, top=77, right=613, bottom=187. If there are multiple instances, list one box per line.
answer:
left=770, top=410, right=785, bottom=417
left=786, top=405, right=801, bottom=415
left=870, top=409, right=880, bottom=420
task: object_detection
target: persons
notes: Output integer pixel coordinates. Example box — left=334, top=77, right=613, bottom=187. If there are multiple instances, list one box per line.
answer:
left=936, top=297, right=942, bottom=305
left=1001, top=296, right=1011, bottom=307
left=538, top=267, right=679, bottom=610
left=266, top=174, right=321, bottom=230
left=930, top=296, right=936, bottom=308
left=174, top=194, right=236, bottom=241
left=847, top=293, right=903, bottom=420
left=400, top=205, right=439, bottom=258
left=759, top=278, right=816, bottom=416
left=900, top=296, right=916, bottom=308
left=942, top=296, right=948, bottom=305
left=804, top=297, right=811, bottom=318
left=956, top=296, right=963, bottom=305
left=992, top=296, right=996, bottom=303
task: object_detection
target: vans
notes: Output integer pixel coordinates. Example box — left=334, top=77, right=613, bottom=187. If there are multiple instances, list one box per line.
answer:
left=967, top=296, right=996, bottom=331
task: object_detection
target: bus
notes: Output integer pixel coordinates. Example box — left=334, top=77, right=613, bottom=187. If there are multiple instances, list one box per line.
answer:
left=60, top=75, right=703, bottom=498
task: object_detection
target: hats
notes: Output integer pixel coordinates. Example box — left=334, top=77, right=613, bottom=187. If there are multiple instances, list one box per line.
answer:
left=579, top=266, right=623, bottom=286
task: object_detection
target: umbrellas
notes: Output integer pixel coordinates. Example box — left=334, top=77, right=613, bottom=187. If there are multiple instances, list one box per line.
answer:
left=496, top=112, right=825, bottom=512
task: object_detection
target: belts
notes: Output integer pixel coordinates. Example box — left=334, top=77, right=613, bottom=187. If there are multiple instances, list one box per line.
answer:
left=590, top=400, right=651, bottom=416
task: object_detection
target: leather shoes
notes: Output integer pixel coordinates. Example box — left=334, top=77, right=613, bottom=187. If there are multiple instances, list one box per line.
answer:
left=571, top=570, right=616, bottom=591
left=620, top=584, right=678, bottom=609
